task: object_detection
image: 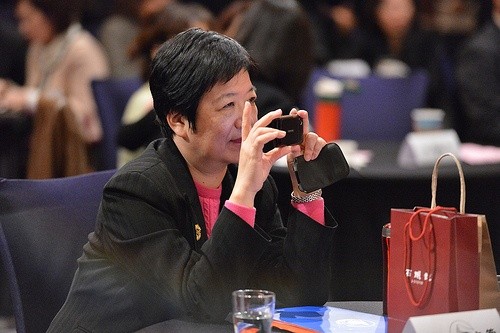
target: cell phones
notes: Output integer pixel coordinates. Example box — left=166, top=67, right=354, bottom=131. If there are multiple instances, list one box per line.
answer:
left=270, top=116, right=304, bottom=148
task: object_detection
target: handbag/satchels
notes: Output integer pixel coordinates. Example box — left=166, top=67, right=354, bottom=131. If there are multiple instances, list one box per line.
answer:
left=386, top=206, right=479, bottom=333
left=431, top=151, right=499, bottom=312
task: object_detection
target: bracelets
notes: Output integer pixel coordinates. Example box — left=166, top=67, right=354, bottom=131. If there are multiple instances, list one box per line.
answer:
left=290, top=188, right=322, bottom=204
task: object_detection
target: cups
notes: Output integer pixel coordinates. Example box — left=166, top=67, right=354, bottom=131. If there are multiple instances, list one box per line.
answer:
left=410, top=109, right=446, bottom=133
left=314, top=77, right=342, bottom=145
left=232, top=289, right=275, bottom=333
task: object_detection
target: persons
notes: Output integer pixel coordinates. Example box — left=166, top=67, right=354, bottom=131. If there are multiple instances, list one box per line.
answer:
left=45, top=27, right=340, bottom=332
left=0, top=0, right=500, bottom=181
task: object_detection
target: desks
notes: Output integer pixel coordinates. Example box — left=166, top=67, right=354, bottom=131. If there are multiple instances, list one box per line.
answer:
left=130, top=308, right=384, bottom=333
left=264, top=137, right=500, bottom=180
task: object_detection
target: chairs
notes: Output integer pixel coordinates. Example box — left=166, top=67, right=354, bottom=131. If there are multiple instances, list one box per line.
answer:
left=0, top=170, right=117, bottom=333
left=89, top=74, right=142, bottom=169
left=304, top=68, right=426, bottom=140
